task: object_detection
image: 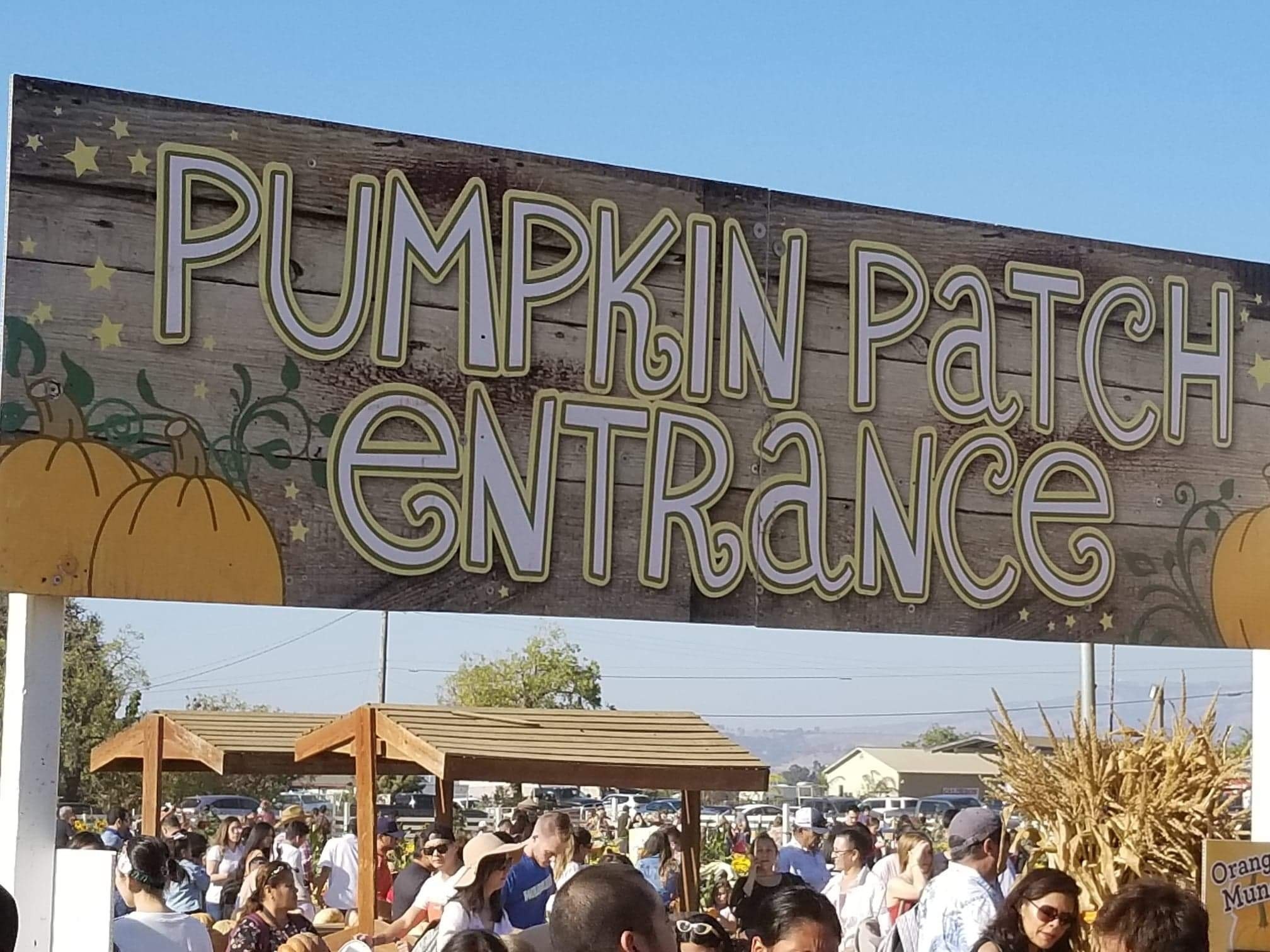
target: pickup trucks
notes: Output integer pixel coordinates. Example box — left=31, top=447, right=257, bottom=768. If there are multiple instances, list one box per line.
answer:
left=349, top=791, right=456, bottom=837
left=789, top=796, right=861, bottom=818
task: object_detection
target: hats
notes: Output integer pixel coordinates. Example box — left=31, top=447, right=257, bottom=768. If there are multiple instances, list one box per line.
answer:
left=623, top=805, right=631, bottom=811
left=377, top=817, right=406, bottom=839
left=792, top=807, right=830, bottom=835
left=273, top=805, right=313, bottom=829
left=454, top=833, right=529, bottom=892
left=948, top=806, right=1020, bottom=853
left=422, top=825, right=456, bottom=845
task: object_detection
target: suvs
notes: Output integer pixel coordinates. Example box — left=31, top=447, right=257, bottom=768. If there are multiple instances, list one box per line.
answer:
left=601, top=793, right=652, bottom=822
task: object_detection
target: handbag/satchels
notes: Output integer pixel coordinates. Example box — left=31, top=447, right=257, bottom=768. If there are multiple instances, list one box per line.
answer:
left=220, top=880, right=242, bottom=906
left=411, top=897, right=471, bottom=952
left=668, top=873, right=685, bottom=914
left=853, top=876, right=904, bottom=952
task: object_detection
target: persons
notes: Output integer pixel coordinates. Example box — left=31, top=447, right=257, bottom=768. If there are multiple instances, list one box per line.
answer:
left=100, top=807, right=130, bottom=851
left=972, top=867, right=1082, bottom=952
left=56, top=806, right=76, bottom=848
left=355, top=808, right=594, bottom=952
left=66, top=832, right=106, bottom=849
left=550, top=863, right=679, bottom=952
left=111, top=836, right=214, bottom=952
left=163, top=800, right=358, bottom=952
left=714, top=804, right=1016, bottom=952
left=1092, top=878, right=1208, bottom=952
left=375, top=817, right=405, bottom=903
left=636, top=825, right=681, bottom=909
left=675, top=914, right=733, bottom=952
left=587, top=802, right=648, bottom=837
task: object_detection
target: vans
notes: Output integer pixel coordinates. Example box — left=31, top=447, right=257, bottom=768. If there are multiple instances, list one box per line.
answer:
left=276, top=791, right=333, bottom=814
left=914, top=794, right=984, bottom=820
left=178, top=795, right=279, bottom=820
left=856, top=796, right=918, bottom=822
left=306, top=788, right=350, bottom=809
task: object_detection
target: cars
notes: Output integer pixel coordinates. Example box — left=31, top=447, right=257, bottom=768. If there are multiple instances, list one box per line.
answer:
left=699, top=806, right=732, bottom=832
left=640, top=799, right=705, bottom=815
left=981, top=795, right=1022, bottom=828
left=452, top=786, right=603, bottom=831
left=723, top=804, right=795, bottom=830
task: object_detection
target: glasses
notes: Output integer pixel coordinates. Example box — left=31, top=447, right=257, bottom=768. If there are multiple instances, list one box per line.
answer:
left=423, top=842, right=451, bottom=855
left=982, top=833, right=1011, bottom=847
left=121, top=817, right=130, bottom=823
left=1024, top=895, right=1074, bottom=929
left=676, top=920, right=720, bottom=939
left=832, top=847, right=852, bottom=854
left=264, top=863, right=291, bottom=886
left=496, top=857, right=514, bottom=871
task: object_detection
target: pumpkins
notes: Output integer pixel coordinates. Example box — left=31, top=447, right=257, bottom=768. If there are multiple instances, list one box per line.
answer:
left=275, top=932, right=331, bottom=952
left=313, top=907, right=345, bottom=924
left=189, top=912, right=237, bottom=952
